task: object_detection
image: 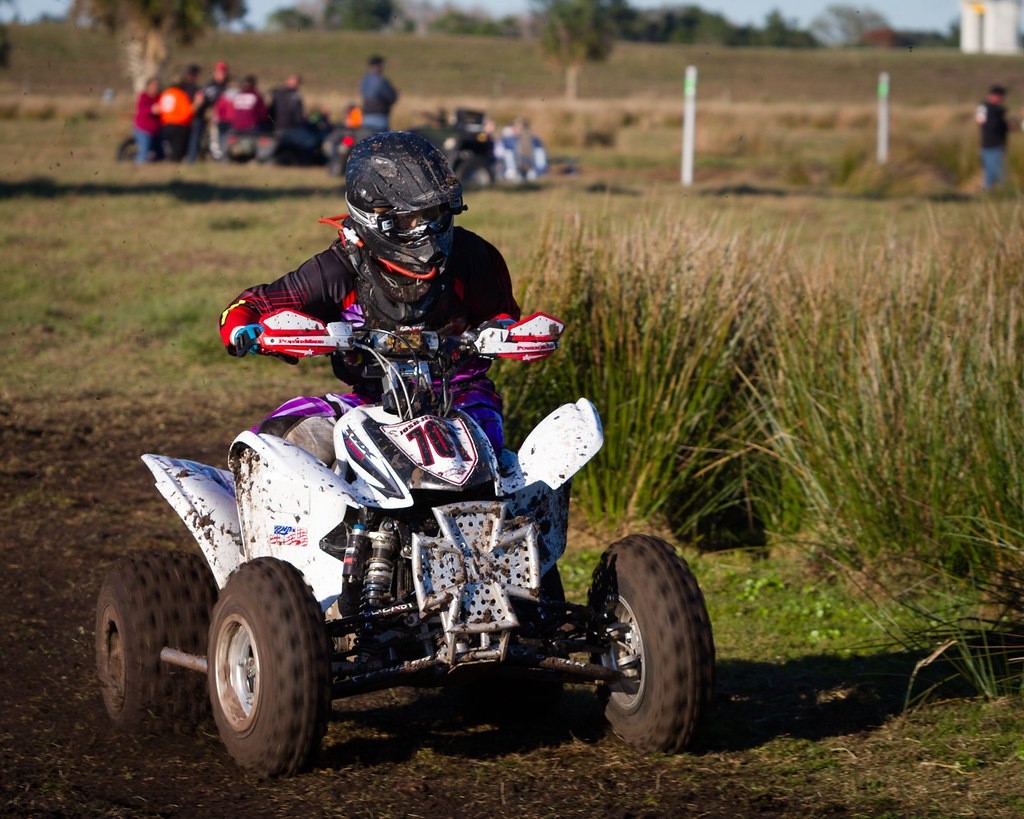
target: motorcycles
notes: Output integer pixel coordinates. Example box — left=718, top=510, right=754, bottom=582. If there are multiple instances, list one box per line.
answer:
left=95, top=308, right=718, bottom=783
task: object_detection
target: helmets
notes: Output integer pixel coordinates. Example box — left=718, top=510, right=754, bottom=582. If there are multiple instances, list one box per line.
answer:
left=346, top=131, right=463, bottom=301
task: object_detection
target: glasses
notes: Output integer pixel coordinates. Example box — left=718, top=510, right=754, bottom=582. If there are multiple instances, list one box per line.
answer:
left=345, top=191, right=454, bottom=242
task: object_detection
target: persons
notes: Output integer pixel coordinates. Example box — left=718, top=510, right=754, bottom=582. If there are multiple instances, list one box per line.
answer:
left=131, top=51, right=399, bottom=175
left=974, top=84, right=1022, bottom=193
left=219, top=131, right=523, bottom=490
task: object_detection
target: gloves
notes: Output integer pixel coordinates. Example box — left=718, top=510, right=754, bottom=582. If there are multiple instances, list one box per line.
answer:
left=494, top=320, right=517, bottom=331
left=227, top=325, right=264, bottom=355
